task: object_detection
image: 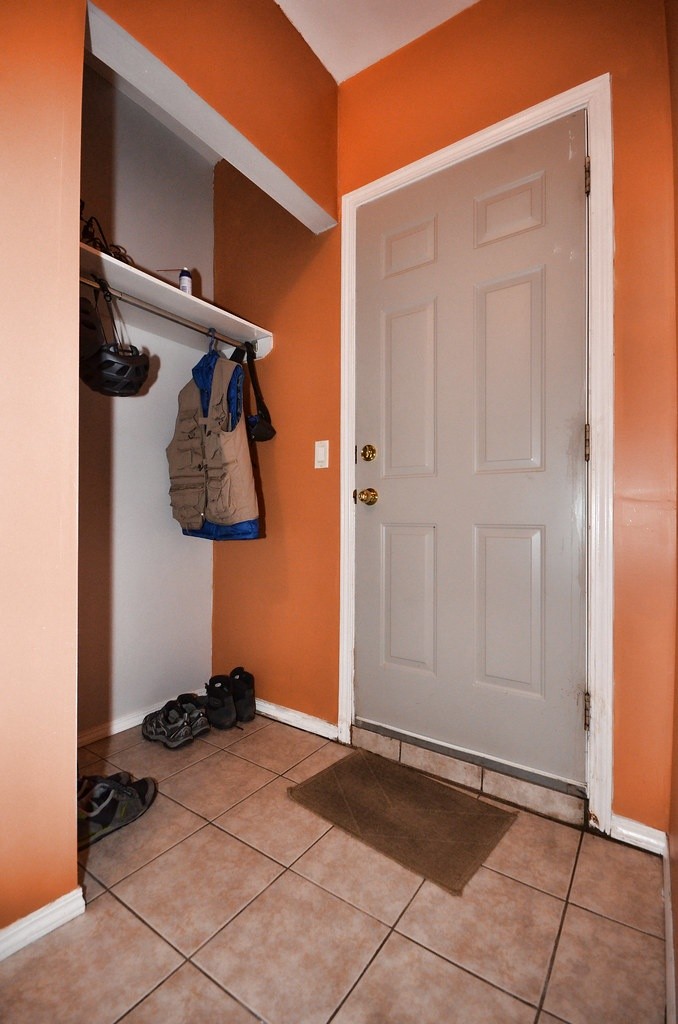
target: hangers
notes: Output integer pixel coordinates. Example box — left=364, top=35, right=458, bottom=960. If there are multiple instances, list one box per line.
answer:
left=206, top=328, right=215, bottom=356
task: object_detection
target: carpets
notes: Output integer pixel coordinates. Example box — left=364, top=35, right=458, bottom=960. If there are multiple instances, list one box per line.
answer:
left=288, top=748, right=519, bottom=898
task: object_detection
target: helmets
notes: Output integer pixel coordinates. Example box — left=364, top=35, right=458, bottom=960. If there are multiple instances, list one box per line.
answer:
left=80, top=342, right=150, bottom=397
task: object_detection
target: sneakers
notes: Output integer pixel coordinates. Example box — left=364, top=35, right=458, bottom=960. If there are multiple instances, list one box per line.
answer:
left=77, top=770, right=159, bottom=852
left=141, top=693, right=211, bottom=748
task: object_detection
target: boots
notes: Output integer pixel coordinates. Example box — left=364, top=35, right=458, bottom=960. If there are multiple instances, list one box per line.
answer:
left=194, top=666, right=257, bottom=731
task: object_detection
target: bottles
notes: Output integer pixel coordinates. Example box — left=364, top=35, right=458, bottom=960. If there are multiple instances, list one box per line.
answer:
left=178, top=266, right=192, bottom=296
